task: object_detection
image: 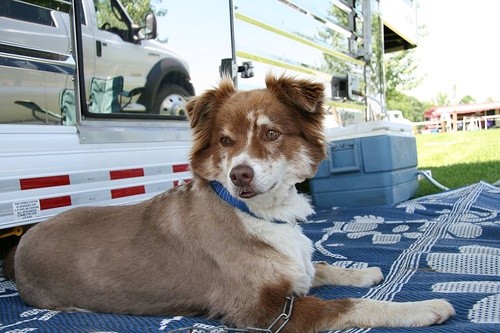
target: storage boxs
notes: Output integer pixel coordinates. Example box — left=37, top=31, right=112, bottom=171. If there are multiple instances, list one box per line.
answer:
left=309, top=111, right=419, bottom=208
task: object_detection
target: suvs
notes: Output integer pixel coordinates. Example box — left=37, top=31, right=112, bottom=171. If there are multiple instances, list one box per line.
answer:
left=0, top=0, right=196, bottom=127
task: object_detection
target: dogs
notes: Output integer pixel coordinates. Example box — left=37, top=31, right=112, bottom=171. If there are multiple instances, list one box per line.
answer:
left=3, top=68, right=457, bottom=333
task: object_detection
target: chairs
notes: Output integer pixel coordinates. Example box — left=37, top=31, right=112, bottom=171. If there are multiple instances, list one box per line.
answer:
left=14, top=77, right=145, bottom=126
